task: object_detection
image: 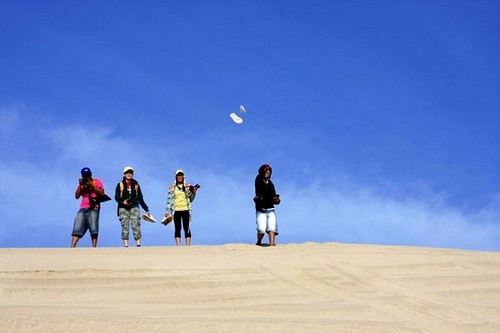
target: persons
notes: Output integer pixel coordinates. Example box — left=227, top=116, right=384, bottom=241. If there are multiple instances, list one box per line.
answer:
left=253, top=163, right=281, bottom=246
left=71, top=166, right=104, bottom=247
left=115, top=166, right=151, bottom=247
left=165, top=169, right=198, bottom=246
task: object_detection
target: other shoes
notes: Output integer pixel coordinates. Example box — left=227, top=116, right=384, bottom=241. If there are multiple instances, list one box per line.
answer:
left=142, top=214, right=156, bottom=222
left=160, top=215, right=173, bottom=225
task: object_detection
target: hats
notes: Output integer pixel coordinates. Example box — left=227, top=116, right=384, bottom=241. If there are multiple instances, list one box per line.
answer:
left=124, top=166, right=134, bottom=173
left=81, top=167, right=91, bottom=175
left=176, top=170, right=184, bottom=175
